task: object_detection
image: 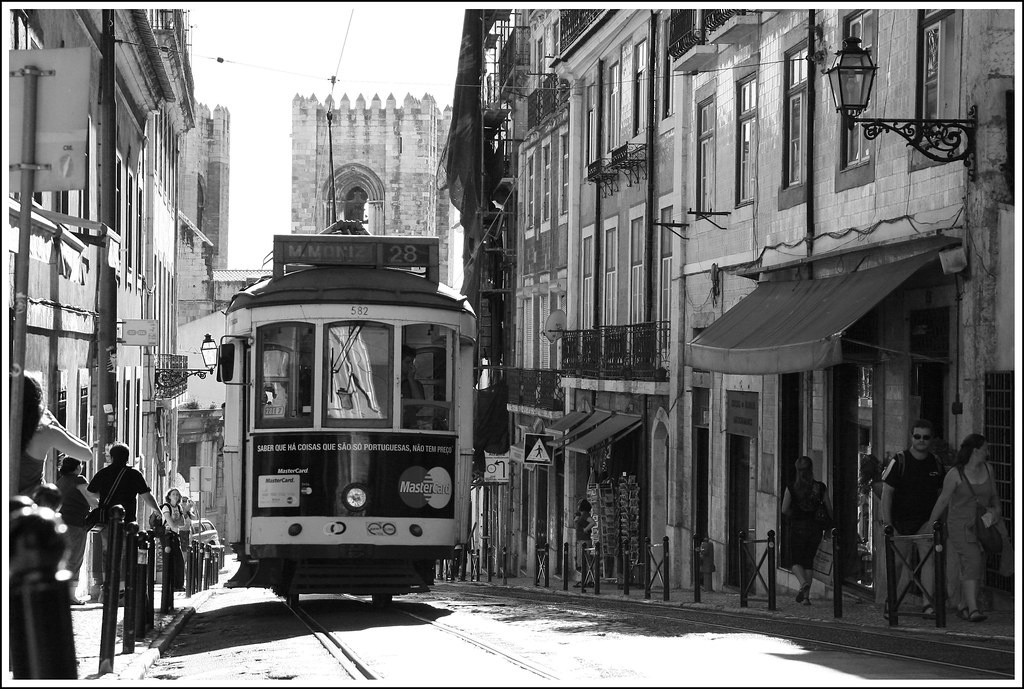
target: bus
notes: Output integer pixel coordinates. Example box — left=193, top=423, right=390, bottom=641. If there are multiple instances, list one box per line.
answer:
left=213, top=197, right=479, bottom=610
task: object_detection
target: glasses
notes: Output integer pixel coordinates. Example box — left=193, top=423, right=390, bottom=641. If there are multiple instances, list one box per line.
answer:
left=181, top=500, right=187, bottom=502
left=912, top=434, right=933, bottom=441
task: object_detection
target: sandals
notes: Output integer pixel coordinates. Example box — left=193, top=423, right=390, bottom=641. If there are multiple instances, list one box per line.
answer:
left=956, top=605, right=970, bottom=619
left=883, top=598, right=889, bottom=620
left=573, top=582, right=584, bottom=588
left=796, top=582, right=810, bottom=603
left=589, top=582, right=594, bottom=588
left=967, top=609, right=987, bottom=622
left=922, top=604, right=937, bottom=620
left=802, top=597, right=811, bottom=605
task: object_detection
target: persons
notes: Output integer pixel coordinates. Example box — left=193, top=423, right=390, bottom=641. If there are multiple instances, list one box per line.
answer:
left=56, top=455, right=100, bottom=605
left=86, top=443, right=163, bottom=600
left=160, top=489, right=191, bottom=591
left=880, top=419, right=946, bottom=620
left=399, top=344, right=425, bottom=429
left=924, top=434, right=1003, bottom=622
left=9, top=374, right=92, bottom=500
left=179, top=496, right=197, bottom=552
left=573, top=499, right=595, bottom=588
left=781, top=455, right=835, bottom=606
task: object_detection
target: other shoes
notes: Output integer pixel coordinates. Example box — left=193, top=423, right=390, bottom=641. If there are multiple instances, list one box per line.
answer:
left=70, top=597, right=85, bottom=605
left=172, top=586, right=186, bottom=591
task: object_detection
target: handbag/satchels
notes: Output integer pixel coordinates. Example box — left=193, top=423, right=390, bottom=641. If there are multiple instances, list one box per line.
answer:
left=976, top=508, right=1003, bottom=556
left=85, top=507, right=108, bottom=534
left=811, top=528, right=839, bottom=585
left=816, top=482, right=830, bottom=530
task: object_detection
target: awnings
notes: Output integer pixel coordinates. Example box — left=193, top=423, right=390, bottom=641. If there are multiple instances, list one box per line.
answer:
left=684, top=250, right=952, bottom=374
left=545, top=410, right=613, bottom=447
left=33, top=207, right=122, bottom=269
left=564, top=410, right=644, bottom=454
left=544, top=411, right=589, bottom=436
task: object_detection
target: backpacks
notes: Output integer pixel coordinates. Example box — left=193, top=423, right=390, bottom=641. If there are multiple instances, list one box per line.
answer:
left=148, top=503, right=181, bottom=538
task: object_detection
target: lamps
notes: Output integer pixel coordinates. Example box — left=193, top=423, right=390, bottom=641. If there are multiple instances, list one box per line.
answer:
left=820, top=35, right=978, bottom=184
left=153, top=333, right=218, bottom=397
